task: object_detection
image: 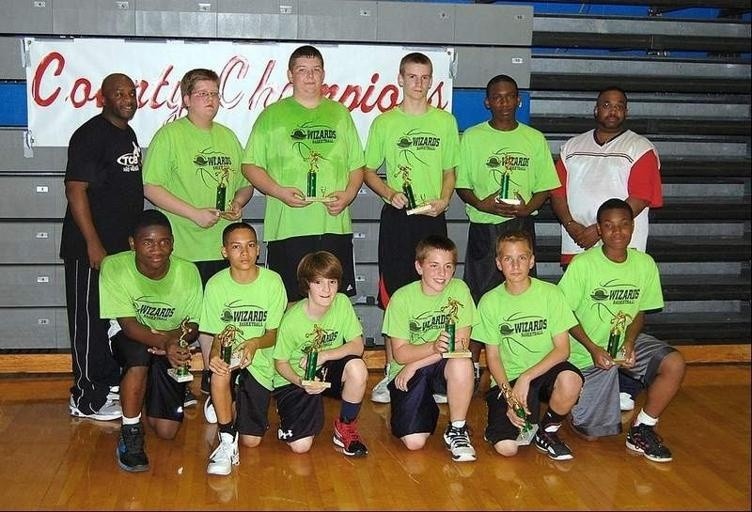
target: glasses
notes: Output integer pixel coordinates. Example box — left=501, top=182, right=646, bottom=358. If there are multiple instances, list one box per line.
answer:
left=187, top=90, right=220, bottom=98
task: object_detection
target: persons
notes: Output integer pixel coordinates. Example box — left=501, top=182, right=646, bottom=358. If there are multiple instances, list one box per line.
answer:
left=381, top=234, right=480, bottom=462
left=241, top=45, right=366, bottom=306
left=469, top=230, right=585, bottom=461
left=141, top=69, right=254, bottom=409
left=363, top=52, right=463, bottom=405
left=454, top=75, right=562, bottom=394
left=556, top=198, right=683, bottom=462
left=272, top=251, right=370, bottom=457
left=549, top=84, right=663, bottom=411
left=99, top=209, right=203, bottom=472
left=59, top=73, right=145, bottom=421
left=199, top=222, right=288, bottom=475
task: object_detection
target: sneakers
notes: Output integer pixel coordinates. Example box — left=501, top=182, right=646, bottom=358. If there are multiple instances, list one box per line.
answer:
left=371, top=363, right=391, bottom=403
left=184, top=385, right=198, bottom=408
left=625, top=418, right=673, bottom=462
left=444, top=420, right=476, bottom=462
left=207, top=430, right=240, bottom=476
left=203, top=370, right=241, bottom=424
left=69, top=399, right=122, bottom=420
left=533, top=422, right=574, bottom=460
left=333, top=415, right=368, bottom=457
left=115, top=420, right=150, bottom=472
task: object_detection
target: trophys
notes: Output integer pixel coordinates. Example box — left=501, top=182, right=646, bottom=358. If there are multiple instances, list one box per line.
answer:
left=495, top=156, right=523, bottom=205
left=299, top=323, right=331, bottom=388
left=209, top=165, right=238, bottom=218
left=167, top=316, right=194, bottom=382
left=440, top=298, right=474, bottom=359
left=392, top=164, right=436, bottom=217
left=606, top=311, right=628, bottom=364
left=498, top=382, right=539, bottom=444
left=217, top=325, right=248, bottom=370
left=301, top=152, right=338, bottom=203
